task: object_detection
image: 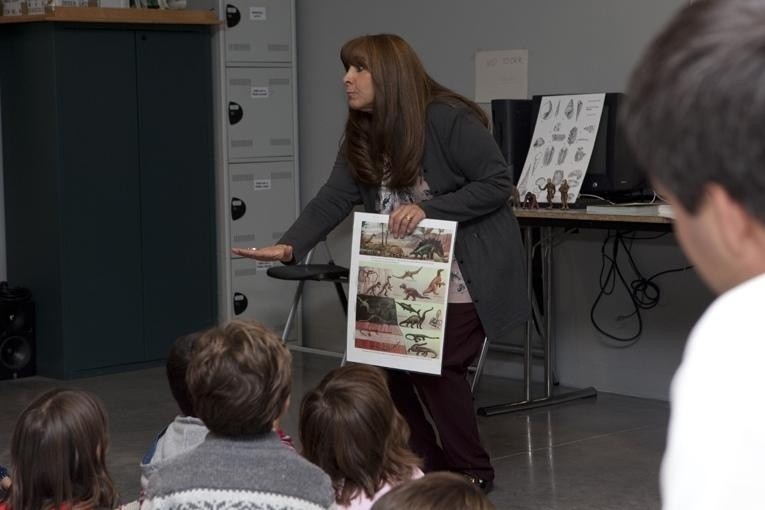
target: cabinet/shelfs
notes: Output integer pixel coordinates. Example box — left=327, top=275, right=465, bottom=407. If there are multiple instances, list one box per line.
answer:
left=217, top=1, right=304, bottom=360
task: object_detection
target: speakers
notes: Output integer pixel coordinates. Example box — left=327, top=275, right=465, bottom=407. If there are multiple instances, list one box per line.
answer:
left=0, top=281, right=36, bottom=381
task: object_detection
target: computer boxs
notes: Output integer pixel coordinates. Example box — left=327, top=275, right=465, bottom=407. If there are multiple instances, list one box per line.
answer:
left=491, top=99, right=532, bottom=187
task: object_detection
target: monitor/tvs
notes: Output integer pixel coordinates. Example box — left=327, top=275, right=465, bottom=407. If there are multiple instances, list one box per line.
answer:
left=532, top=93, right=650, bottom=200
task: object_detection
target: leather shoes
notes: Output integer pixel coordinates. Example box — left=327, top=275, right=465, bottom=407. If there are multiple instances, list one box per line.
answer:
left=461, top=473, right=494, bottom=496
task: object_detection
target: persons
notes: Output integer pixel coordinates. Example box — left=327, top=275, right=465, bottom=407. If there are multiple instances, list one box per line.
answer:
left=135, top=317, right=336, bottom=510
left=0, top=385, right=119, bottom=508
left=136, top=330, right=212, bottom=491
left=368, top=470, right=498, bottom=510
left=228, top=31, right=533, bottom=495
left=292, top=360, right=428, bottom=510
left=616, top=0, right=764, bottom=509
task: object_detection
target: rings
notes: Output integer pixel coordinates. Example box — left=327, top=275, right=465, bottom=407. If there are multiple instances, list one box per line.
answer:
left=404, top=215, right=413, bottom=222
left=247, top=248, right=257, bottom=251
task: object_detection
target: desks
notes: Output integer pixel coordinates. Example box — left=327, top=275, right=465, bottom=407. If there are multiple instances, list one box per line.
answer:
left=476, top=202, right=675, bottom=418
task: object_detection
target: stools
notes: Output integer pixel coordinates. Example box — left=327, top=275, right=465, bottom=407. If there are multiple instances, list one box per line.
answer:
left=265, top=235, right=350, bottom=369
